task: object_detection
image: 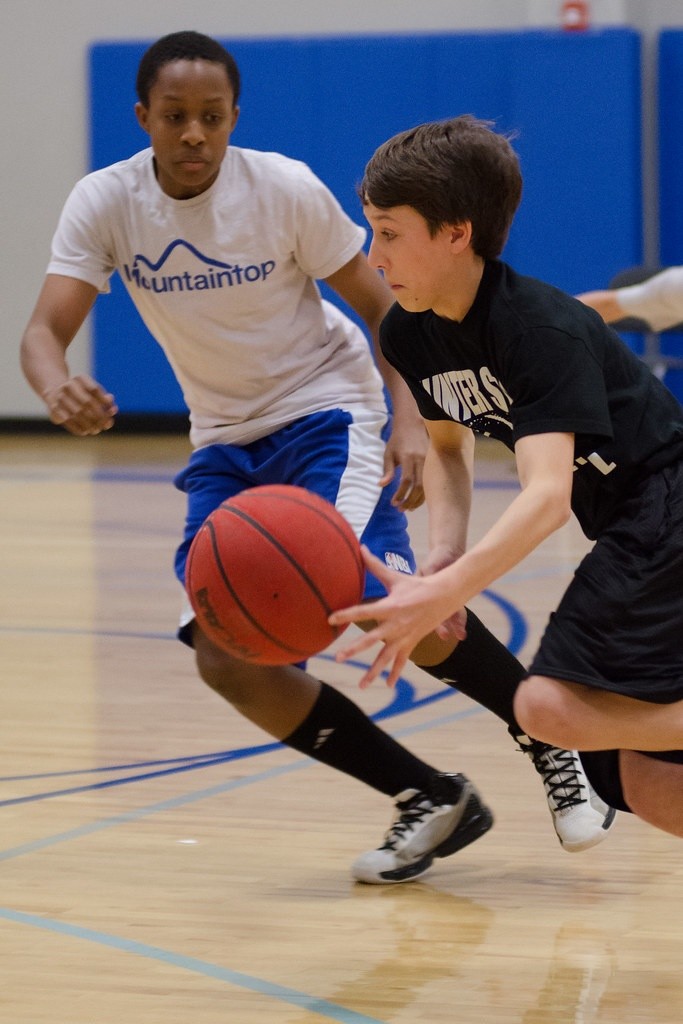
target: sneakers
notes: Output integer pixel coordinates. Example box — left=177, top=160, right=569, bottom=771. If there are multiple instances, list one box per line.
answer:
left=507, top=717, right=615, bottom=851
left=351, top=774, right=493, bottom=883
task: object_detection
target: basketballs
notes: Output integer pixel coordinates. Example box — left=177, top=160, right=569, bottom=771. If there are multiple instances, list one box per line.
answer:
left=185, top=483, right=365, bottom=669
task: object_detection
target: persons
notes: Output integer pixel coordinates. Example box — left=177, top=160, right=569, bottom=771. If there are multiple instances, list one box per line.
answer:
left=329, top=119, right=683, bottom=837
left=18, top=30, right=616, bottom=885
left=575, top=267, right=683, bottom=334
